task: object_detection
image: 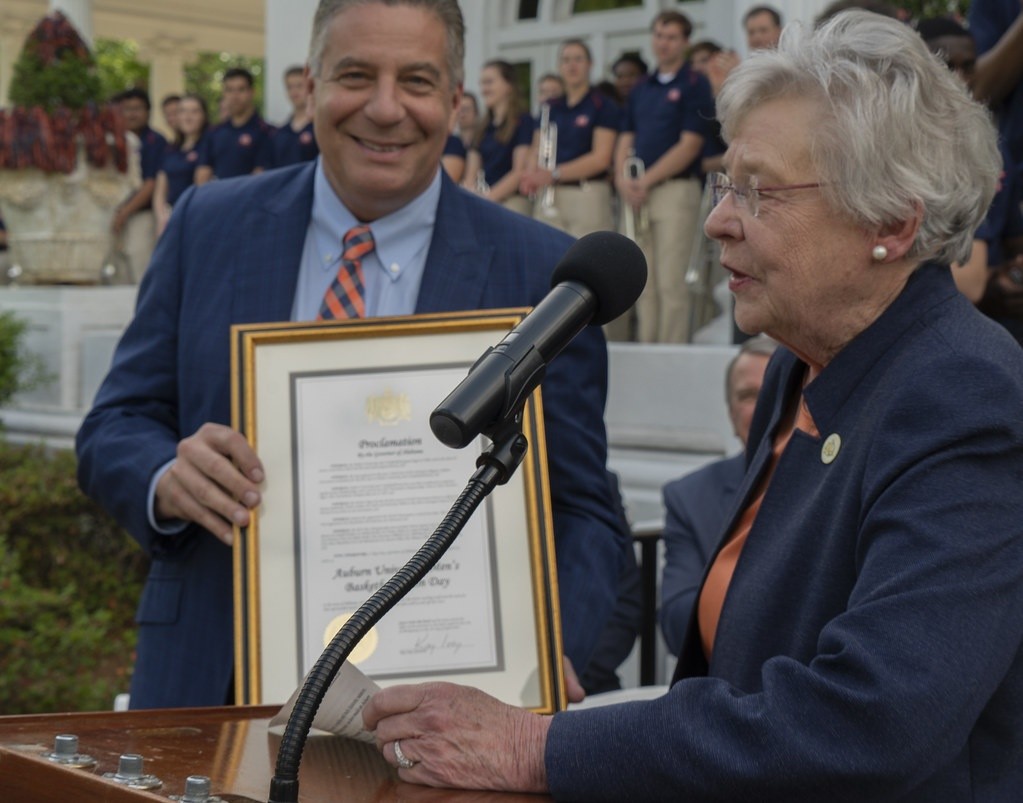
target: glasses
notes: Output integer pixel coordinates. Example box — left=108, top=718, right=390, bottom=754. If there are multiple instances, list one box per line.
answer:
left=708, top=172, right=839, bottom=217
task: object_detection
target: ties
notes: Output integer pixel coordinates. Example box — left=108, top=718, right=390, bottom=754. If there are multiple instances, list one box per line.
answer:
left=314, top=224, right=376, bottom=321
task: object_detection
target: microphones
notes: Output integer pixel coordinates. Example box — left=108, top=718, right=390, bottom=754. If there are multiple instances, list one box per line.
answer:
left=429, top=230, right=648, bottom=450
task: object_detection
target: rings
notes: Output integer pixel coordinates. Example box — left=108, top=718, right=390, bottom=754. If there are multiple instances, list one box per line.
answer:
left=394, top=741, right=414, bottom=768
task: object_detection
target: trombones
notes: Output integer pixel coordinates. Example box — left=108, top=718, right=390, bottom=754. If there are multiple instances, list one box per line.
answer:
left=533, top=103, right=557, bottom=224
left=622, top=154, right=662, bottom=342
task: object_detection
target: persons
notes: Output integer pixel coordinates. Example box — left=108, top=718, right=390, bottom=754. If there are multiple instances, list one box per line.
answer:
left=658, top=336, right=779, bottom=658
left=76, top=0, right=640, bottom=713
left=362, top=7, right=1023, bottom=803
left=111, top=0, right=1022, bottom=347
